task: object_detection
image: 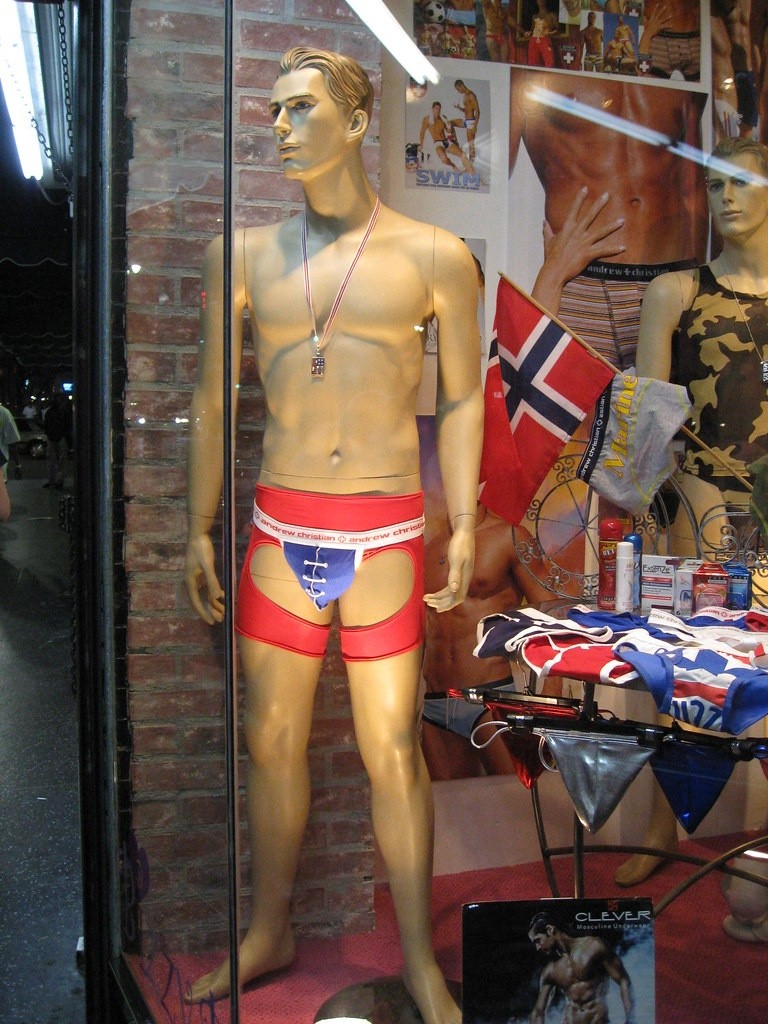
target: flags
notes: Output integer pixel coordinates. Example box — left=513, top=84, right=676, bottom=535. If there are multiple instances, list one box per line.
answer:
left=479, top=278, right=616, bottom=529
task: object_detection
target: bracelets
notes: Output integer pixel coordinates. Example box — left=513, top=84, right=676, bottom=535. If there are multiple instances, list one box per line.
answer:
left=16, top=464, right=22, bottom=468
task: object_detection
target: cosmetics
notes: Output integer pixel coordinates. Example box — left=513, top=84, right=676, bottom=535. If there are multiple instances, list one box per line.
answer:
left=597, top=519, right=642, bottom=615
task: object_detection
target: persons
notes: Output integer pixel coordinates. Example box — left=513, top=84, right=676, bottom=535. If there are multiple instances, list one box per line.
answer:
left=417, top=101, right=488, bottom=187
left=525, top=0, right=560, bottom=68
left=0, top=397, right=72, bottom=521
left=709, top=0, right=768, bottom=148
left=413, top=0, right=477, bottom=60
left=636, top=134, right=768, bottom=610
left=449, top=79, right=480, bottom=164
left=638, top=0, right=700, bottom=83
left=510, top=67, right=708, bottom=376
left=525, top=910, right=635, bottom=1024
left=420, top=496, right=566, bottom=785
left=559, top=0, right=644, bottom=76
left=481, top=0, right=530, bottom=63
left=179, top=46, right=485, bottom=1024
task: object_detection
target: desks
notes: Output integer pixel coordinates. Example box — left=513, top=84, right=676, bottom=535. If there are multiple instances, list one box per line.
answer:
left=468, top=676, right=768, bottom=923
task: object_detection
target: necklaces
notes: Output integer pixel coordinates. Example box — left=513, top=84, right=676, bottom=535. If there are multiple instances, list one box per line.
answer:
left=300, top=192, right=380, bottom=377
left=438, top=510, right=488, bottom=565
left=719, top=251, right=768, bottom=384
